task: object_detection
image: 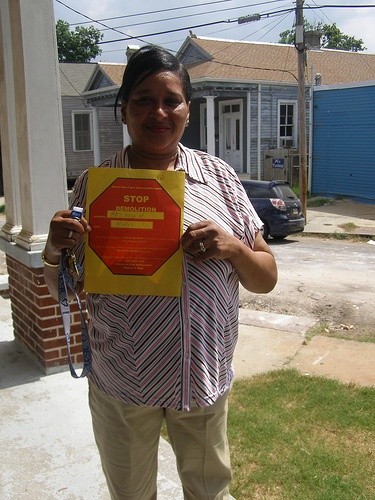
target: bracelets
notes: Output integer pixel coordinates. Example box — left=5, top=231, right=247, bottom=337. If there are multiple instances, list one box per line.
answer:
left=41, top=250, right=61, bottom=267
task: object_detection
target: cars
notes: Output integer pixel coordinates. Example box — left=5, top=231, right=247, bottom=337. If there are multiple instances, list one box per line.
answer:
left=240, top=178, right=305, bottom=243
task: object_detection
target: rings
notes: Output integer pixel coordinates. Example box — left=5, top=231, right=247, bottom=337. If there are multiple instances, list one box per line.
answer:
left=68, top=231, right=72, bottom=238
left=199, top=241, right=206, bottom=252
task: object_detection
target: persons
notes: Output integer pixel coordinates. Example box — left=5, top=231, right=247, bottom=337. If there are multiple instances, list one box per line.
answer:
left=43, top=46, right=277, bottom=500
